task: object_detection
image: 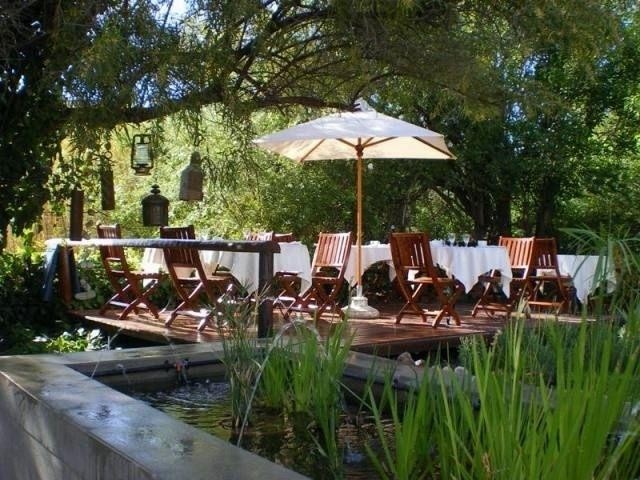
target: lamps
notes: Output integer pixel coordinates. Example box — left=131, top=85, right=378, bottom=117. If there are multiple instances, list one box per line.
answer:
left=129, top=121, right=155, bottom=175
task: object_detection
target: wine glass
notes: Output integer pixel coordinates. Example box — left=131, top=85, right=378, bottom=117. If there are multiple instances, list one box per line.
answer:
left=462, top=234, right=470, bottom=247
left=448, top=232, right=456, bottom=248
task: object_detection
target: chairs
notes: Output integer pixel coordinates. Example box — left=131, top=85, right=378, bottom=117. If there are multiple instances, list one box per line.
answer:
left=387, top=232, right=464, bottom=328
left=467, top=235, right=537, bottom=328
left=94, top=224, right=242, bottom=330
left=278, top=233, right=354, bottom=328
left=527, top=237, right=572, bottom=317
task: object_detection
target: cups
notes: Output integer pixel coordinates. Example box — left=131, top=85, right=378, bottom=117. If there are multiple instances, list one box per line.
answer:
left=243, top=228, right=251, bottom=241
left=259, top=231, right=266, bottom=243
left=477, top=240, right=488, bottom=248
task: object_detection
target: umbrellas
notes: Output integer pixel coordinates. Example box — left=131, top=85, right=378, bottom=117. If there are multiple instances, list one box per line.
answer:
left=249, top=110, right=458, bottom=286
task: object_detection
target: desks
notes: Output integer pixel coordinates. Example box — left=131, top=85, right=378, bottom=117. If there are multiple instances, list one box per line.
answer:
left=537, top=252, right=618, bottom=295
left=228, top=235, right=312, bottom=308
